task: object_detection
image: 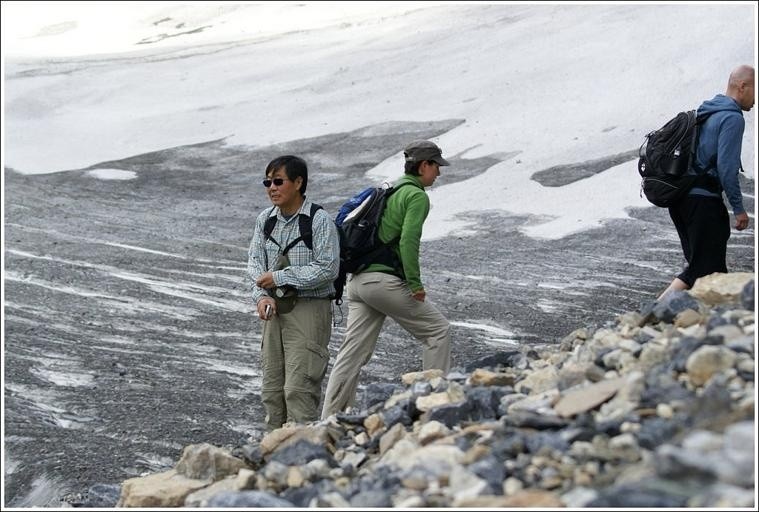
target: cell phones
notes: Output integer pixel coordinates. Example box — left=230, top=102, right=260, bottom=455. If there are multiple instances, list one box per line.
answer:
left=265, top=304, right=272, bottom=320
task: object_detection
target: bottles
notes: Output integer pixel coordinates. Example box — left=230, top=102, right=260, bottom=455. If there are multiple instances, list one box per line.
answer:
left=667, top=150, right=681, bottom=176
left=357, top=218, right=369, bottom=228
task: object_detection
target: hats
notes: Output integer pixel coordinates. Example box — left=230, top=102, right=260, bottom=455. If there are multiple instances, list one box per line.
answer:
left=404, top=141, right=450, bottom=168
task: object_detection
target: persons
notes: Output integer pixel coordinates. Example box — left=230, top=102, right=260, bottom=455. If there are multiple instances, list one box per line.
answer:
left=639, top=66, right=755, bottom=304
left=248, top=155, right=340, bottom=434
left=320, top=140, right=451, bottom=420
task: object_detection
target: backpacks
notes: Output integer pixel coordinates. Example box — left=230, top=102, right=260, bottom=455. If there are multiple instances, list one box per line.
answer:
left=636, top=108, right=744, bottom=209
left=264, top=203, right=349, bottom=305
left=335, top=179, right=425, bottom=274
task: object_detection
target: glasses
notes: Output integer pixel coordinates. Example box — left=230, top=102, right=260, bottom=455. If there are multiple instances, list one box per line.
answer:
left=262, top=178, right=290, bottom=187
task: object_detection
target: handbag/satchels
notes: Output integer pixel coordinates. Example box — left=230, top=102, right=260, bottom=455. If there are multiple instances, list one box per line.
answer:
left=267, top=254, right=298, bottom=314
